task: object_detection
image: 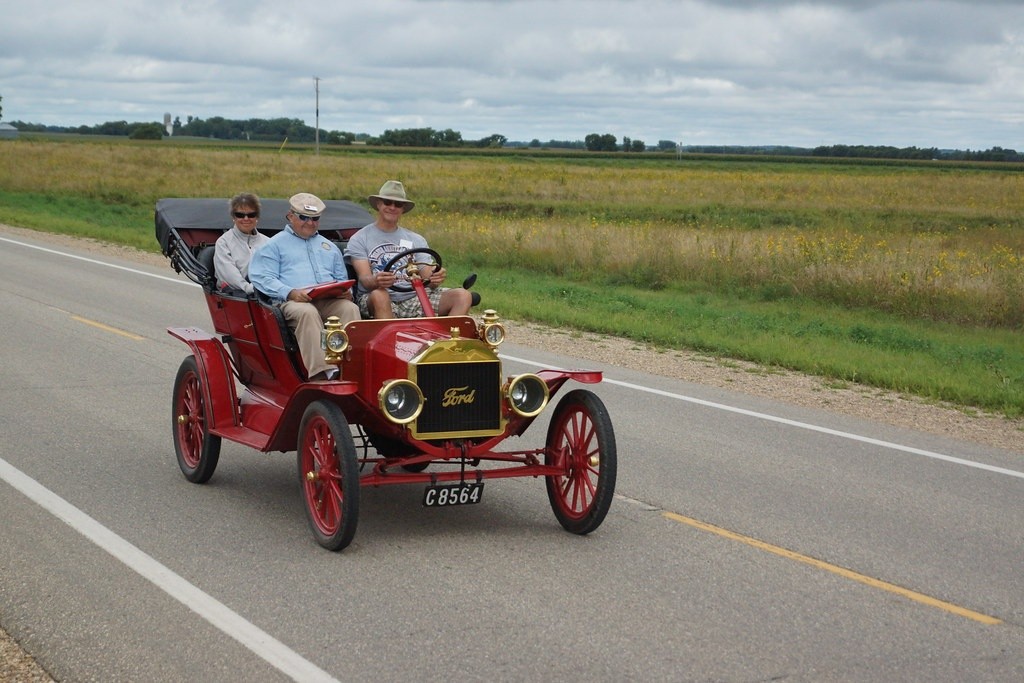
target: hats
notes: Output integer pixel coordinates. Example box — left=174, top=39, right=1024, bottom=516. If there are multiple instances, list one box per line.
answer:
left=289, top=193, right=326, bottom=217
left=368, top=181, right=415, bottom=214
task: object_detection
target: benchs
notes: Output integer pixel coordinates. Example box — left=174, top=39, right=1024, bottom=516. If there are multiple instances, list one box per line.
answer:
left=197, top=239, right=367, bottom=357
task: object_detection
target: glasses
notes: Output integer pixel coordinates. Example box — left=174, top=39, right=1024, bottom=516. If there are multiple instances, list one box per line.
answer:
left=380, top=198, right=406, bottom=208
left=234, top=212, right=257, bottom=218
left=293, top=213, right=320, bottom=222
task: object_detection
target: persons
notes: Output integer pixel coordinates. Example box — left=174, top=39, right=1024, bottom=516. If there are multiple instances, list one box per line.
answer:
left=247, top=192, right=362, bottom=381
left=213, top=192, right=270, bottom=299
left=343, top=180, right=473, bottom=319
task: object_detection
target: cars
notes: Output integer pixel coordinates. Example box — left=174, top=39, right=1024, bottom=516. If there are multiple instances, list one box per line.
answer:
left=151, top=197, right=618, bottom=550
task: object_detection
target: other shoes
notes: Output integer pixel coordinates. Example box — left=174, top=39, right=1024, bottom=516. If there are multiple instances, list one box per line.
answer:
left=324, top=368, right=340, bottom=381
